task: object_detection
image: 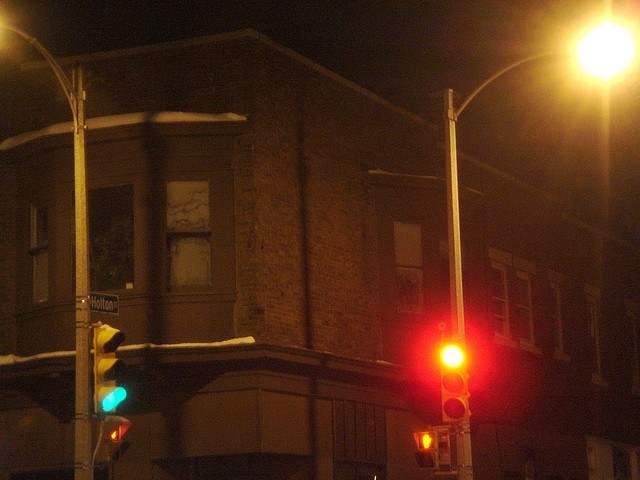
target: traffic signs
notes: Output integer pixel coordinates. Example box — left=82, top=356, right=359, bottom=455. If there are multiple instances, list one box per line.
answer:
left=90, top=292, right=119, bottom=314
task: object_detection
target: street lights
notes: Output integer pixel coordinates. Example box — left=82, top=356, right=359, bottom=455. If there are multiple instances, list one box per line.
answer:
left=446, top=26, right=632, bottom=480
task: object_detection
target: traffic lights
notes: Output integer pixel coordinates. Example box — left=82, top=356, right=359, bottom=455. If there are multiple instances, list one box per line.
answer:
left=438, top=338, right=468, bottom=423
left=414, top=431, right=439, bottom=470
left=93, top=323, right=128, bottom=414
left=92, top=416, right=131, bottom=462
